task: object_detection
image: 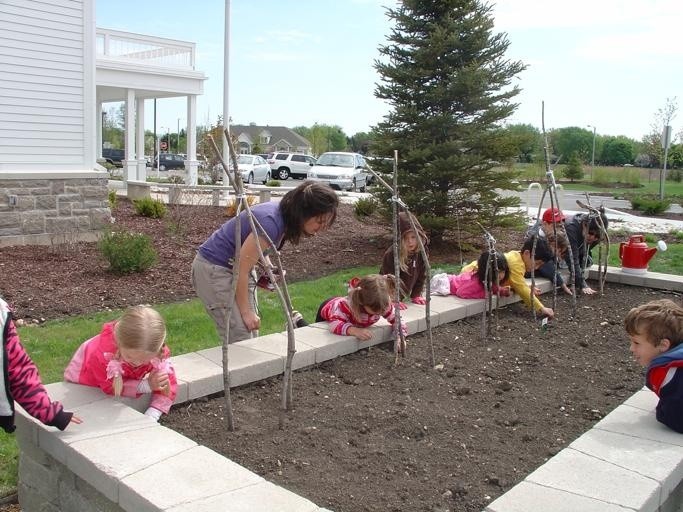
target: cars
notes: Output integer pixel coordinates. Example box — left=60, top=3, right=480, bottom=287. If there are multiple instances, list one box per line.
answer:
left=307, top=151, right=368, bottom=192
left=368, top=155, right=429, bottom=180
left=155, top=151, right=207, bottom=171
left=215, top=152, right=272, bottom=183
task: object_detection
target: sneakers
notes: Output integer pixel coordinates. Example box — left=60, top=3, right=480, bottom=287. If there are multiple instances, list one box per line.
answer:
left=285, top=310, right=302, bottom=331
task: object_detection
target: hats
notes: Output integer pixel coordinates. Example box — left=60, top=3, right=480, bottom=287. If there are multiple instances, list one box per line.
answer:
left=542, top=207, right=568, bottom=223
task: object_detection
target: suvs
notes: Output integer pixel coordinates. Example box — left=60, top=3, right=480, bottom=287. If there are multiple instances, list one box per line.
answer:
left=103, top=149, right=149, bottom=168
left=266, top=151, right=317, bottom=179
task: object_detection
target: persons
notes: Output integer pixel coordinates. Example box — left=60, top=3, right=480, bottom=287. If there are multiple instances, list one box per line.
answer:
left=563, top=203, right=608, bottom=297
left=417, top=248, right=510, bottom=300
left=458, top=233, right=554, bottom=321
left=0, top=296, right=85, bottom=434
left=622, top=297, right=682, bottom=437
left=377, top=210, right=431, bottom=310
left=62, top=304, right=180, bottom=420
left=519, top=207, right=569, bottom=269
left=190, top=180, right=340, bottom=345
left=283, top=271, right=410, bottom=353
left=522, top=230, right=573, bottom=296
